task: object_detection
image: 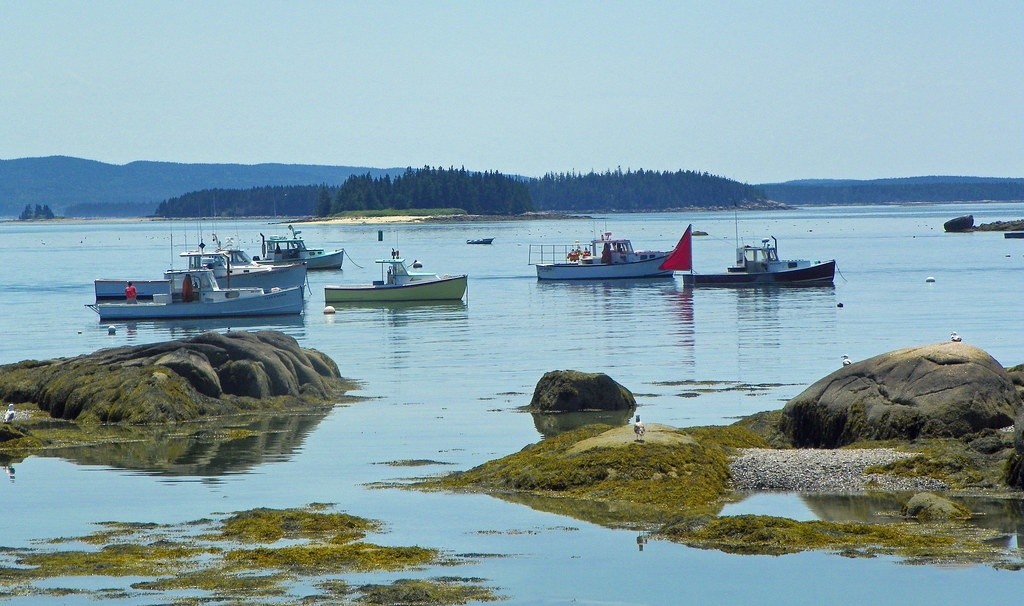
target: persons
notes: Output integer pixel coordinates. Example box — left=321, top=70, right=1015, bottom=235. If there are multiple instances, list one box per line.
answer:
left=183, top=274, right=194, bottom=302
left=125, top=281, right=137, bottom=304
left=567, top=248, right=591, bottom=262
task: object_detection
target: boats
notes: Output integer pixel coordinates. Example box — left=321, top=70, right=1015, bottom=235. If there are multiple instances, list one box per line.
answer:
left=1004, top=232, right=1024, bottom=238
left=98, top=268, right=307, bottom=321
left=467, top=238, right=495, bottom=244
left=535, top=230, right=676, bottom=280
left=323, top=257, right=469, bottom=304
left=95, top=244, right=309, bottom=298
left=673, top=236, right=836, bottom=287
left=251, top=224, right=344, bottom=270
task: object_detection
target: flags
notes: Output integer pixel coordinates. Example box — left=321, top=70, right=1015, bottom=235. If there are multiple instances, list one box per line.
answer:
left=658, top=225, right=692, bottom=270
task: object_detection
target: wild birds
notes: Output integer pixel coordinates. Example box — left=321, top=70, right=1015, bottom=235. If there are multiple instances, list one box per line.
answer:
left=2, top=403, right=15, bottom=423
left=634, top=414, right=645, bottom=438
left=951, top=332, right=962, bottom=342
left=841, top=354, right=851, bottom=366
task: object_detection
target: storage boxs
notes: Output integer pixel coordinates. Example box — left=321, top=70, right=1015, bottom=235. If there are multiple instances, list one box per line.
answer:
left=373, top=281, right=384, bottom=285
left=153, top=294, right=171, bottom=303
